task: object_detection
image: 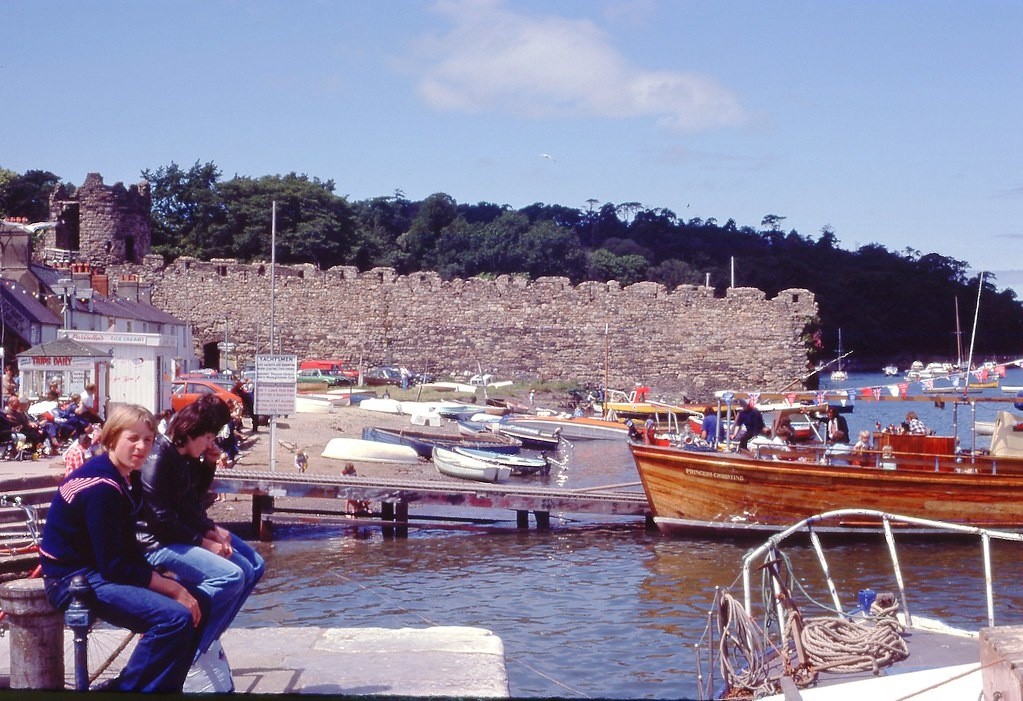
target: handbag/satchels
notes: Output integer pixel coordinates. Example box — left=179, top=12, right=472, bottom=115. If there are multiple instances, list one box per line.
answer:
left=182, top=640, right=234, bottom=694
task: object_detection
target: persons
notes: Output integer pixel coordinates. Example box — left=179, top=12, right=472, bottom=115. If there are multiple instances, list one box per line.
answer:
left=539, top=425, right=564, bottom=461
left=196, top=394, right=266, bottom=694
left=344, top=497, right=372, bottom=536
left=39, top=403, right=212, bottom=694
left=294, top=448, right=309, bottom=473
left=0, top=364, right=261, bottom=504
left=643, top=397, right=928, bottom=466
left=341, top=463, right=356, bottom=478
left=136, top=402, right=256, bottom=694
left=528, top=382, right=605, bottom=416
left=400, top=365, right=411, bottom=390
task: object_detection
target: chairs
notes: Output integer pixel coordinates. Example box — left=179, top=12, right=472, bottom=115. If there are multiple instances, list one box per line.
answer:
left=0, top=420, right=19, bottom=460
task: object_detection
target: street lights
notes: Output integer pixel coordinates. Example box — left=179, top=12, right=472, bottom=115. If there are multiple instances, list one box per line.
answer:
left=58, top=279, right=73, bottom=329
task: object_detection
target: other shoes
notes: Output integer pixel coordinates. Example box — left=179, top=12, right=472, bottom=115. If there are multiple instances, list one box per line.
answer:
left=36, top=443, right=46, bottom=448
left=52, top=441, right=62, bottom=447
left=39, top=454, right=46, bottom=458
left=98, top=679, right=114, bottom=692
left=53, top=449, right=58, bottom=454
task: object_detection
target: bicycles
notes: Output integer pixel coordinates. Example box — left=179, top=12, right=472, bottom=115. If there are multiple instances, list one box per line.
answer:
left=0, top=495, right=99, bottom=637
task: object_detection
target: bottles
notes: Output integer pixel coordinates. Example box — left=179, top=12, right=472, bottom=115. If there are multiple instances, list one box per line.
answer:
left=876, top=422, right=902, bottom=434
left=33, top=452, right=38, bottom=461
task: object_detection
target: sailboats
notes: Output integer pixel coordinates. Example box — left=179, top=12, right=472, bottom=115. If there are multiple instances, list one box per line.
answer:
left=956, top=269, right=983, bottom=405
left=831, top=328, right=848, bottom=381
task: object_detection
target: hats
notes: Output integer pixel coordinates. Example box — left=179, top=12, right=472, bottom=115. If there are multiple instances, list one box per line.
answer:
left=834, top=431, right=845, bottom=441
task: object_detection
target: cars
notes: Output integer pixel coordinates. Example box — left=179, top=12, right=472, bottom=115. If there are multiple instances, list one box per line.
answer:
left=170, top=372, right=254, bottom=412
left=294, top=361, right=435, bottom=386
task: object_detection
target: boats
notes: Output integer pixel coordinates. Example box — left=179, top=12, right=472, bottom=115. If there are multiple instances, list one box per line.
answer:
left=359, top=398, right=563, bottom=448
left=922, top=387, right=984, bottom=394
left=969, top=380, right=998, bottom=388
left=627, top=390, right=1023, bottom=534
left=593, top=388, right=736, bottom=421
left=979, top=362, right=1005, bottom=379
left=1000, top=386, right=1023, bottom=391
left=975, top=421, right=996, bottom=435
left=362, top=427, right=522, bottom=460
left=432, top=446, right=512, bottom=483
left=885, top=366, right=900, bottom=377
left=903, top=361, right=971, bottom=382
left=321, top=438, right=418, bottom=464
left=454, top=447, right=546, bottom=475
